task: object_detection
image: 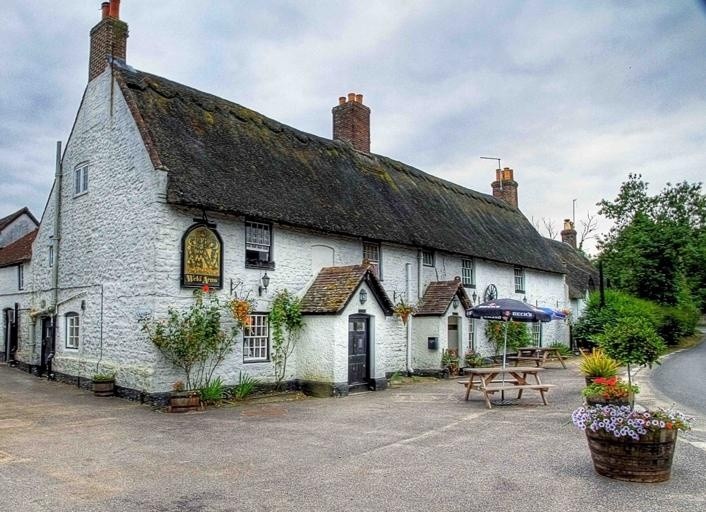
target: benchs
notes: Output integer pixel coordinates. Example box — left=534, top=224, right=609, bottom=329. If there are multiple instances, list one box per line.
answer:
left=526, top=354, right=568, bottom=368
left=458, top=379, right=527, bottom=400
left=507, top=356, right=546, bottom=368
left=481, top=384, right=557, bottom=408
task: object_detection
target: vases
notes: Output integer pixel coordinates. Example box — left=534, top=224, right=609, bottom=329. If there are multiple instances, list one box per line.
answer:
left=586, top=397, right=630, bottom=409
left=584, top=428, right=678, bottom=483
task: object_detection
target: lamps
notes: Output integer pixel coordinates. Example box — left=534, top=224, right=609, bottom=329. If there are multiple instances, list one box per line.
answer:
left=360, top=289, right=367, bottom=304
left=453, top=297, right=459, bottom=309
left=259, top=273, right=270, bottom=296
left=472, top=292, right=477, bottom=303
left=523, top=296, right=527, bottom=303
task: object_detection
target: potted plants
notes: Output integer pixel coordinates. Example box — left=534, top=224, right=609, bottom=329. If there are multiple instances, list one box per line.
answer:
left=569, top=346, right=623, bottom=387
left=91, top=373, right=116, bottom=397
left=136, top=288, right=224, bottom=411
left=165, top=381, right=190, bottom=412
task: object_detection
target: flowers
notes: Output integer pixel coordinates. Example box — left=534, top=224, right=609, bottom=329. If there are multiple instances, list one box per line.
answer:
left=582, top=376, right=639, bottom=402
left=571, top=404, right=697, bottom=440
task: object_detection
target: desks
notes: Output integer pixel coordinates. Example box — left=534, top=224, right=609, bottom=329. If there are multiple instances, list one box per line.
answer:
left=514, top=348, right=568, bottom=369
left=463, top=367, right=548, bottom=409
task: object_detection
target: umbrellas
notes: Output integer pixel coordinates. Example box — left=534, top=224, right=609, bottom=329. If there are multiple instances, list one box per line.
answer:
left=535, top=306, right=566, bottom=347
left=465, top=297, right=550, bottom=367
